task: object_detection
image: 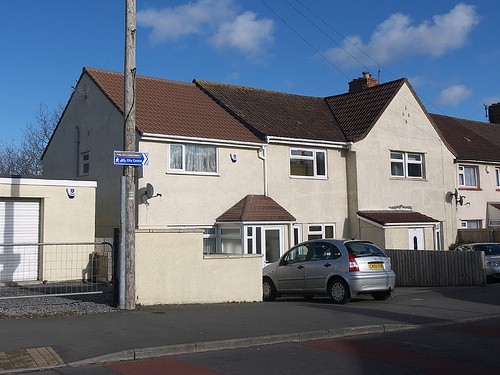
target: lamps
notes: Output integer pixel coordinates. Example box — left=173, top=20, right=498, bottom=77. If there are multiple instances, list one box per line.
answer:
left=457, top=195, right=466, bottom=207
left=449, top=188, right=458, bottom=201
left=139, top=182, right=162, bottom=201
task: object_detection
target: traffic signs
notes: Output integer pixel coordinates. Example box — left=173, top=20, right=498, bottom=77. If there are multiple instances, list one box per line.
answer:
left=114, top=150, right=149, bottom=166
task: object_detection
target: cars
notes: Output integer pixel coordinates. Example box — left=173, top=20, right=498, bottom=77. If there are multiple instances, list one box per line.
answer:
left=262, top=238, right=396, bottom=305
left=445, top=242, right=500, bottom=279
